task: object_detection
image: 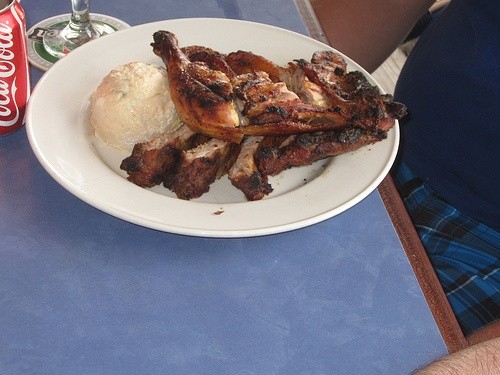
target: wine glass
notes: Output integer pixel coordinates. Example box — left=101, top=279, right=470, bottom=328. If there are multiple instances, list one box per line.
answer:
left=26, top=0, right=132, bottom=72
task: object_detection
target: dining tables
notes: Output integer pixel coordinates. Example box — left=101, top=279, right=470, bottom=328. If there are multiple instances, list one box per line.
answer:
left=0, top=0, right=468, bottom=375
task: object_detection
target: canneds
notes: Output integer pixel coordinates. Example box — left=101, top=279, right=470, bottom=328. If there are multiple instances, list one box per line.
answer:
left=0, top=0, right=32, bottom=135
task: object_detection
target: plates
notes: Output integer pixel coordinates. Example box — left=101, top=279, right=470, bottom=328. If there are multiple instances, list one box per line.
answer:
left=25, top=17, right=401, bottom=240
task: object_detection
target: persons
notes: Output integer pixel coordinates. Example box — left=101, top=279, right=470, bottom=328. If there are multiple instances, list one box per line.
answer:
left=310, top=0, right=500, bottom=375
